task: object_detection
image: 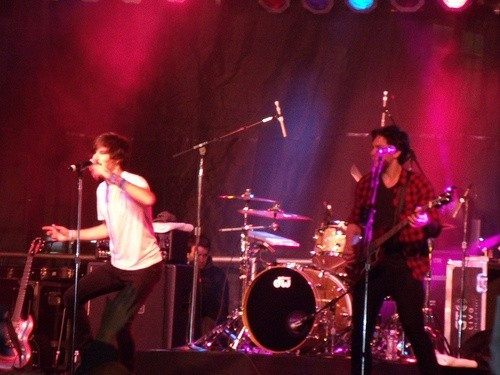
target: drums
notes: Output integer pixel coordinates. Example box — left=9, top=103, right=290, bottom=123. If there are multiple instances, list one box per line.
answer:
left=242, top=263, right=354, bottom=354
left=312, top=220, right=350, bottom=277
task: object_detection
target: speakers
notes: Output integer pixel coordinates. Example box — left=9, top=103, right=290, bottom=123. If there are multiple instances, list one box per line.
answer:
left=445, top=261, right=489, bottom=357
left=200, top=260, right=243, bottom=335
left=83, top=262, right=193, bottom=349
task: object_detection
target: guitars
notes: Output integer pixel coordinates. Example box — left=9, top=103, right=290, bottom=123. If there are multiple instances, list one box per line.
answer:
left=345, top=191, right=452, bottom=277
left=0, top=237, right=45, bottom=373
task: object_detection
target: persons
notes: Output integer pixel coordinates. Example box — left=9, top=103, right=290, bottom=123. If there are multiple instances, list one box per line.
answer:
left=181, top=235, right=229, bottom=348
left=340, top=125, right=448, bottom=375
left=39, top=132, right=167, bottom=375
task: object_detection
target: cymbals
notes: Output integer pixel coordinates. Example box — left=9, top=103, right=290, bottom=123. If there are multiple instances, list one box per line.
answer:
left=247, top=230, right=300, bottom=247
left=237, top=208, right=311, bottom=220
left=218, top=195, right=275, bottom=203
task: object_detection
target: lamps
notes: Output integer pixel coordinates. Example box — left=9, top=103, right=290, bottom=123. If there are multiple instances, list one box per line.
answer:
left=301, top=0, right=335, bottom=15
left=343, top=0, right=378, bottom=14
left=257, top=0, right=291, bottom=15
left=390, top=0, right=425, bottom=13
left=436, top=0, right=472, bottom=13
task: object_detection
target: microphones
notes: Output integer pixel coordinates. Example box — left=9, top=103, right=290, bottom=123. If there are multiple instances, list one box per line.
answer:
left=66, top=159, right=96, bottom=172
left=288, top=317, right=297, bottom=330
left=275, top=101, right=287, bottom=137
left=453, top=186, right=472, bottom=217
